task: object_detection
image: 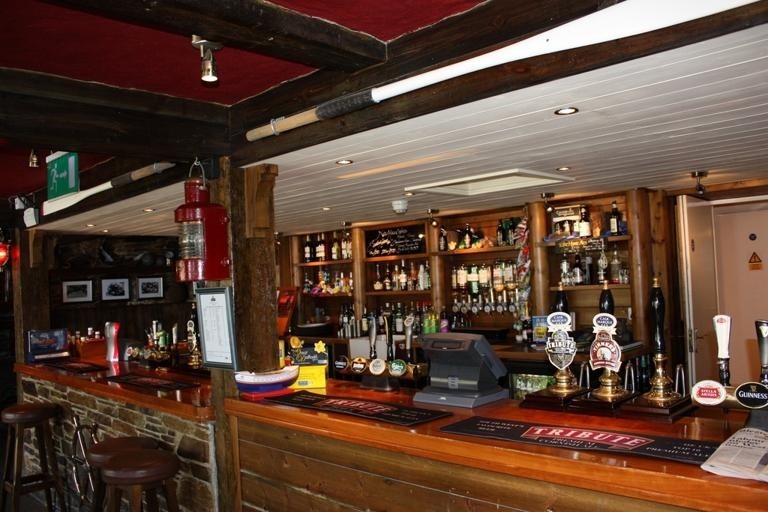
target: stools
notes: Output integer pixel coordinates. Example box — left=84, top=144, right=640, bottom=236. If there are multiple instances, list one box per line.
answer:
left=0, top=402, right=181, bottom=512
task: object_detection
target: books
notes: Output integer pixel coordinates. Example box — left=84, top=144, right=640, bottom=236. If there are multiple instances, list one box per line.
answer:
left=700, top=427, right=768, bottom=483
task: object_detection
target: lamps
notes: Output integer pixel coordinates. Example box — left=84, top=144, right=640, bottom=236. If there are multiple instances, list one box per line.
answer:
left=29, top=147, right=41, bottom=168
left=191, top=34, right=223, bottom=84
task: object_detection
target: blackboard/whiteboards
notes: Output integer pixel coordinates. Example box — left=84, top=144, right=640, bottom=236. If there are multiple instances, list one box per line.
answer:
left=365, top=221, right=430, bottom=261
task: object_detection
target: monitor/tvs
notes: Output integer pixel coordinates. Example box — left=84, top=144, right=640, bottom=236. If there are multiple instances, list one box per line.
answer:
left=422, top=333, right=508, bottom=377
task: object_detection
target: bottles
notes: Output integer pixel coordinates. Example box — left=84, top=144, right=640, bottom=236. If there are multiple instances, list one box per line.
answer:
left=142, top=326, right=171, bottom=353
left=66, top=327, right=100, bottom=348
left=362, top=259, right=435, bottom=336
left=436, top=213, right=531, bottom=342
left=559, top=246, right=629, bottom=285
left=555, top=220, right=579, bottom=239
left=297, top=232, right=355, bottom=324
left=578, top=205, right=592, bottom=239
left=187, top=301, right=199, bottom=342
left=609, top=199, right=622, bottom=235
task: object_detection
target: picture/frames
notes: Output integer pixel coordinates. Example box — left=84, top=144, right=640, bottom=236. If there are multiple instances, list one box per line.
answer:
left=100, top=278, right=131, bottom=302
left=195, top=286, right=238, bottom=369
left=60, top=278, right=94, bottom=304
left=135, top=276, right=164, bottom=300
